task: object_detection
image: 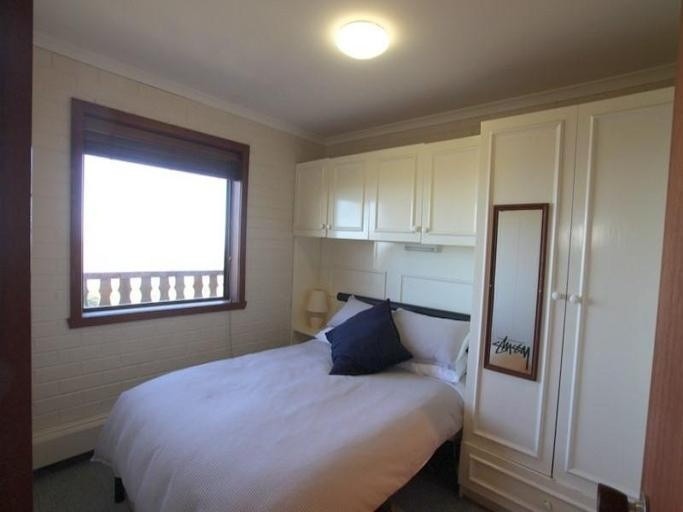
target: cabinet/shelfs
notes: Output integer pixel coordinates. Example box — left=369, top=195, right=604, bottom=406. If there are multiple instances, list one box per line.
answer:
left=369, top=133, right=480, bottom=246
left=294, top=152, right=369, bottom=240
left=456, top=88, right=680, bottom=508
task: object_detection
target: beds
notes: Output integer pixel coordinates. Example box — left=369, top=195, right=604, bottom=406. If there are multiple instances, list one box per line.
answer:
left=88, top=291, right=475, bottom=512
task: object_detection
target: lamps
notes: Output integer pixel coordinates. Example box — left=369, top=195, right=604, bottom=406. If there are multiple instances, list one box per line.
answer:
left=335, top=19, right=391, bottom=61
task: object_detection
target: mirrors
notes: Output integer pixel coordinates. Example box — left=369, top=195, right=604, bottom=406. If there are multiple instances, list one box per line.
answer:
left=484, top=202, right=551, bottom=382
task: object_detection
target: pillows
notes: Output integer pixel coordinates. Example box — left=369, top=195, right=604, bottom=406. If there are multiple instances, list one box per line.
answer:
left=390, top=307, right=471, bottom=384
left=324, top=298, right=414, bottom=375
left=313, top=294, right=375, bottom=346
